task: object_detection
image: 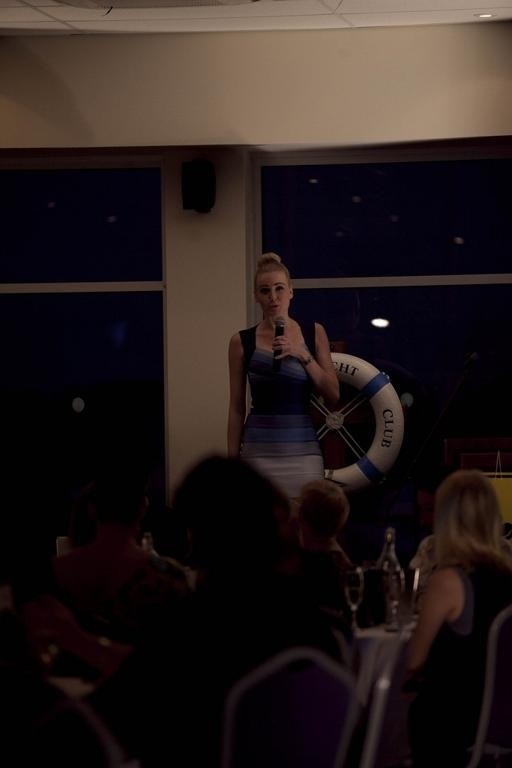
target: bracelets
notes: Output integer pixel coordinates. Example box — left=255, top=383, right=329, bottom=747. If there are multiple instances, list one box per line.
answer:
left=302, top=355, right=314, bottom=366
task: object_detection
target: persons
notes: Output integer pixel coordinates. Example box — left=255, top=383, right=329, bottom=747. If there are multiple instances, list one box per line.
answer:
left=228, top=253, right=340, bottom=497
left=0, top=453, right=511, bottom=767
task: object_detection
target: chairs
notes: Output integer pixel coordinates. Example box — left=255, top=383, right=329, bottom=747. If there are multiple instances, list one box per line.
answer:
left=468, top=608, right=512, bottom=768
left=221, top=643, right=366, bottom=767
left=362, top=629, right=417, bottom=767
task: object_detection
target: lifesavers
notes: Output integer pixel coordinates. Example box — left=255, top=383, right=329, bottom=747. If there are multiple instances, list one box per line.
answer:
left=321, top=353, right=405, bottom=491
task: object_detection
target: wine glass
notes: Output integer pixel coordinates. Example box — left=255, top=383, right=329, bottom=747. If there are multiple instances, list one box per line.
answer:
left=344, top=567, right=364, bottom=636
left=382, top=573, right=402, bottom=633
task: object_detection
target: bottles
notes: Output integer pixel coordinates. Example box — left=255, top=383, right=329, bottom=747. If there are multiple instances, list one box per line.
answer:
left=139, top=532, right=158, bottom=559
left=378, top=528, right=407, bottom=622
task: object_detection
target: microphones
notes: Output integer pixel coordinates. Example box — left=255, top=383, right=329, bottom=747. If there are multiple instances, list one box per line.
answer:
left=273, top=316, right=286, bottom=370
